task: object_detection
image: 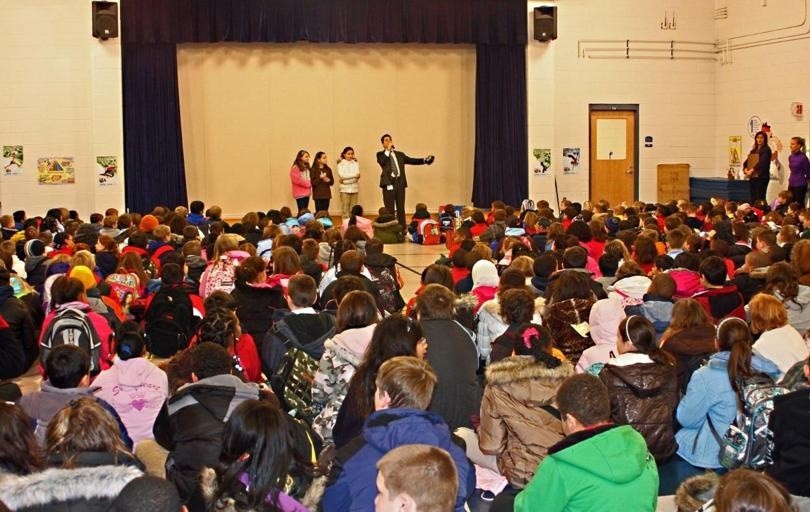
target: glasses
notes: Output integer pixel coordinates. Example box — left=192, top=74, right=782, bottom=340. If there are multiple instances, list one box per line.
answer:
left=417, top=337, right=427, bottom=346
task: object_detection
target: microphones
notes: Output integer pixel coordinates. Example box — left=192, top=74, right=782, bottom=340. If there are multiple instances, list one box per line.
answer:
left=390, top=143, right=396, bottom=151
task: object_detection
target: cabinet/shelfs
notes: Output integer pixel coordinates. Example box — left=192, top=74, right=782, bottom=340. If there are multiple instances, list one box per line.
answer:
left=658, top=164, right=690, bottom=204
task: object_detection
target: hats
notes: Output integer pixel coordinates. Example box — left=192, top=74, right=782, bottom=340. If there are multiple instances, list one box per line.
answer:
left=604, top=217, right=621, bottom=231
left=141, top=215, right=159, bottom=233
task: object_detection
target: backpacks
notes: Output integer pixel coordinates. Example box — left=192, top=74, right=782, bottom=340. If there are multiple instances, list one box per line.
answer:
left=104, top=267, right=143, bottom=319
left=40, top=310, right=100, bottom=379
left=205, top=256, right=239, bottom=302
left=438, top=215, right=457, bottom=240
left=145, top=245, right=174, bottom=279
left=411, top=217, right=441, bottom=245
left=706, top=363, right=793, bottom=473
left=145, top=284, right=193, bottom=356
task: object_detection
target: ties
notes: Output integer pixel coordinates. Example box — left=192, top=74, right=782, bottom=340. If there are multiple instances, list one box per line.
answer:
left=389, top=152, right=398, bottom=180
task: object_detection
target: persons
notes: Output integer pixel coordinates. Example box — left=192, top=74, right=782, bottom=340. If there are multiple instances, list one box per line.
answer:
left=742, top=130, right=771, bottom=207
left=785, top=136, right=809, bottom=208
left=0, top=189, right=809, bottom=510
left=309, top=151, right=336, bottom=212
left=4, top=152, right=23, bottom=169
left=536, top=153, right=548, bottom=174
left=375, top=132, right=435, bottom=231
left=335, top=147, right=362, bottom=216
left=288, top=147, right=313, bottom=212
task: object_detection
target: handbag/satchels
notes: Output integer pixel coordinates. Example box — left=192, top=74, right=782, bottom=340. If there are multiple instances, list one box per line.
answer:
left=271, top=345, right=328, bottom=423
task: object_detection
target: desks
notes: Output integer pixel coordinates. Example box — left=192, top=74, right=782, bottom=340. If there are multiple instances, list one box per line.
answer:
left=688, top=176, right=754, bottom=207
